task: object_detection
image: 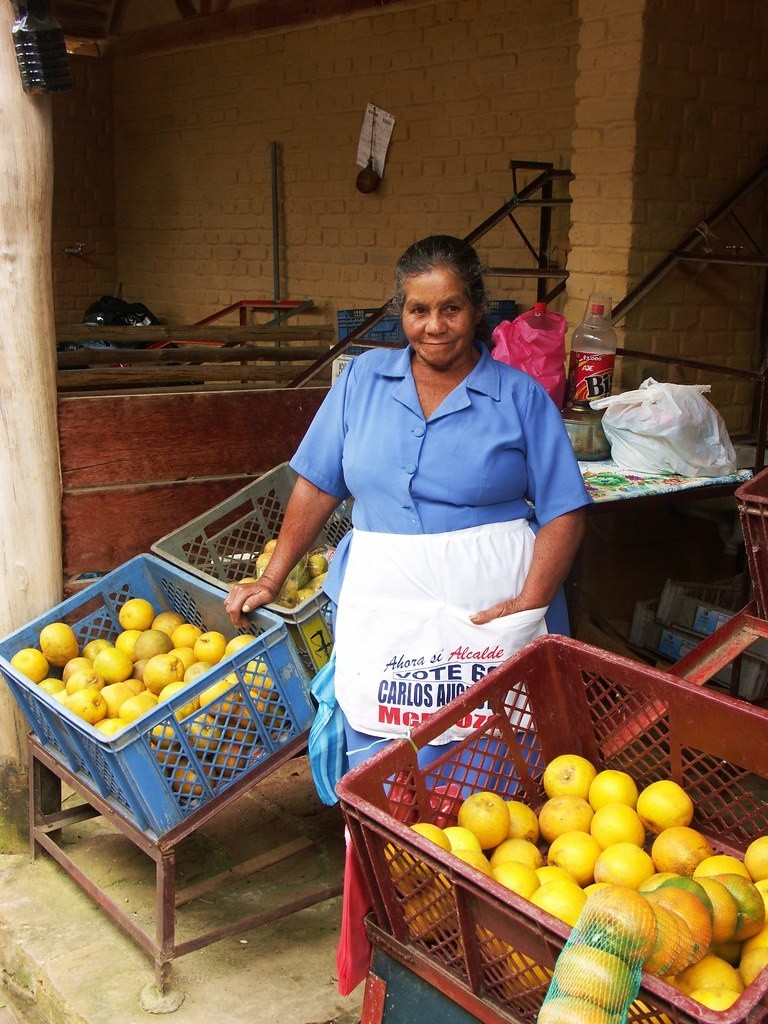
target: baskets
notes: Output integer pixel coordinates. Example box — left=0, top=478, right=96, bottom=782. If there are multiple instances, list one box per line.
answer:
left=336, top=300, right=518, bottom=356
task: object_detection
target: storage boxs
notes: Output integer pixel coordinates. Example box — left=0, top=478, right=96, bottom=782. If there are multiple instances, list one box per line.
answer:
left=0, top=554, right=316, bottom=840
left=148, top=461, right=355, bottom=677
left=338, top=296, right=516, bottom=356
left=577, top=582, right=768, bottom=701
left=735, top=463, right=768, bottom=623
left=334, top=632, right=768, bottom=1024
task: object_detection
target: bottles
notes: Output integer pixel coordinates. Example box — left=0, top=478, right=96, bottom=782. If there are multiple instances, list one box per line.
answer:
left=567, top=306, right=617, bottom=408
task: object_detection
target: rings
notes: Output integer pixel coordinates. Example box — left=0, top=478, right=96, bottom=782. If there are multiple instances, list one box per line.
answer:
left=226, top=601, right=230, bottom=603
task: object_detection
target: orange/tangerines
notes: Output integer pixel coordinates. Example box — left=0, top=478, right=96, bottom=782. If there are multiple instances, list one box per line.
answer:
left=9, top=598, right=289, bottom=801
left=228, top=537, right=329, bottom=608
left=382, top=753, right=768, bottom=1024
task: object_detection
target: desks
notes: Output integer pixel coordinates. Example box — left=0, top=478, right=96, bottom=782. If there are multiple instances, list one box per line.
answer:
left=566, top=455, right=744, bottom=636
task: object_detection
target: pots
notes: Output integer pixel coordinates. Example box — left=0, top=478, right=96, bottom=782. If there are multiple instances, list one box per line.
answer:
left=557, top=399, right=613, bottom=461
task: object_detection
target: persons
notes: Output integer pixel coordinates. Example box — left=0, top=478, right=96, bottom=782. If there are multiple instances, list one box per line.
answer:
left=223, top=234, right=595, bottom=811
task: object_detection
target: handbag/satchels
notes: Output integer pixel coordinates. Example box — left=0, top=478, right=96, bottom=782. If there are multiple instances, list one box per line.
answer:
left=588, top=375, right=738, bottom=479
